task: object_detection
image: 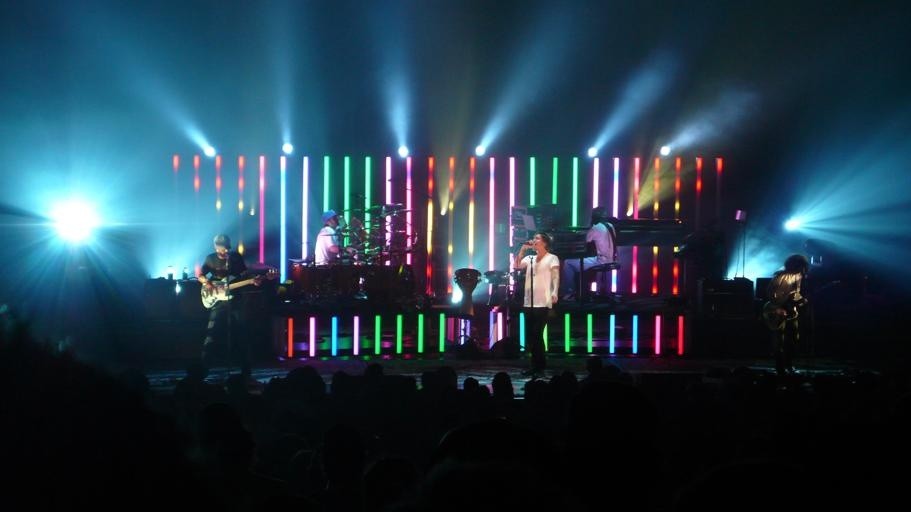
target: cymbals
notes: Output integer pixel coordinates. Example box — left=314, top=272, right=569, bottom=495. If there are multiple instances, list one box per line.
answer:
left=318, top=233, right=355, bottom=238
left=341, top=208, right=361, bottom=213
left=366, top=226, right=388, bottom=234
left=364, top=203, right=403, bottom=216
left=345, top=227, right=366, bottom=232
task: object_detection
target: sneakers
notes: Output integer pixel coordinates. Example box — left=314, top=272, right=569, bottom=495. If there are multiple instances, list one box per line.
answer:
left=563, top=294, right=576, bottom=302
left=522, top=368, right=544, bottom=377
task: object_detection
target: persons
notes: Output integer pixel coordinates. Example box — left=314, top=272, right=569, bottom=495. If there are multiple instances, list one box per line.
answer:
left=314, top=209, right=353, bottom=266
left=560, top=206, right=615, bottom=301
left=199, top=234, right=262, bottom=377
left=766, top=252, right=809, bottom=371
left=513, top=230, right=560, bottom=375
left=2, top=357, right=910, bottom=511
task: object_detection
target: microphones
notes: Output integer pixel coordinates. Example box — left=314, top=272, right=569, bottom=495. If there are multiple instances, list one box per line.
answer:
left=520, top=242, right=533, bottom=245
left=224, top=249, right=228, bottom=256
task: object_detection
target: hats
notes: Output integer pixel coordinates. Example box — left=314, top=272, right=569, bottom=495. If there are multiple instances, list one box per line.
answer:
left=322, top=210, right=337, bottom=225
left=214, top=235, right=230, bottom=248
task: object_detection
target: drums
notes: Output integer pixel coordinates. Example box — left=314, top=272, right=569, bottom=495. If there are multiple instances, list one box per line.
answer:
left=391, top=264, right=417, bottom=295
left=317, top=264, right=360, bottom=301
left=484, top=270, right=519, bottom=307
left=454, top=268, right=481, bottom=317
left=360, top=264, right=392, bottom=301
left=289, top=261, right=319, bottom=299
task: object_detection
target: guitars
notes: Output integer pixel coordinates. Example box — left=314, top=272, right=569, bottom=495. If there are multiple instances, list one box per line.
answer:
left=763, top=280, right=841, bottom=333
left=200, top=268, right=281, bottom=309
